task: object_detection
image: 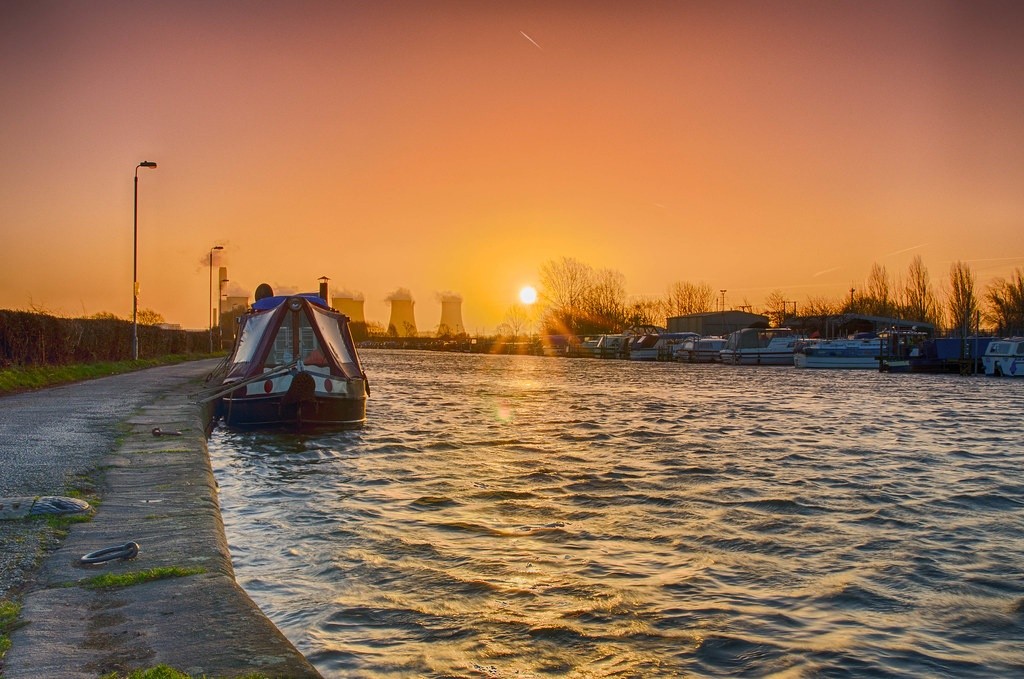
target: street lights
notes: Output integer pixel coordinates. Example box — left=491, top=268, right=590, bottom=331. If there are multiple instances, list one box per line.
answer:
left=219, top=293, right=228, bottom=296
left=719, top=289, right=727, bottom=311
left=850, top=287, right=855, bottom=314
left=131, top=159, right=158, bottom=359
left=220, top=279, right=229, bottom=351
left=209, top=245, right=224, bottom=352
left=231, top=303, right=237, bottom=345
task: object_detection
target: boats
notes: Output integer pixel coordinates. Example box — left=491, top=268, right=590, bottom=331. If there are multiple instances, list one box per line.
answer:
left=352, top=326, right=1024, bottom=376
left=202, top=281, right=371, bottom=433
